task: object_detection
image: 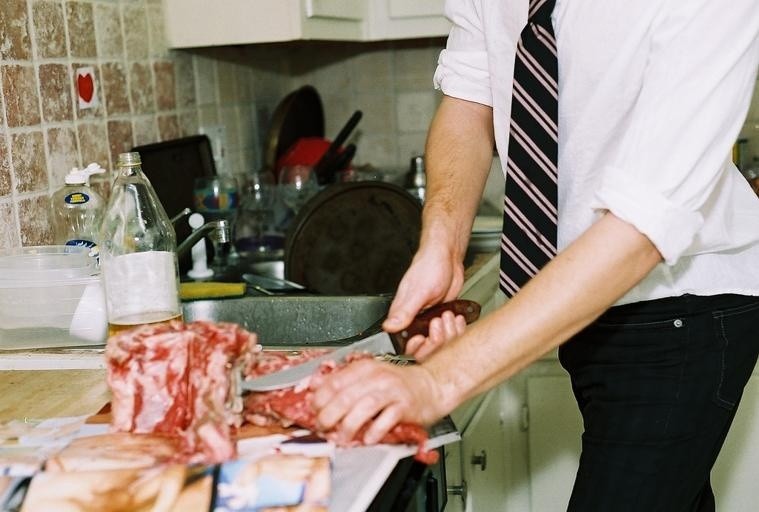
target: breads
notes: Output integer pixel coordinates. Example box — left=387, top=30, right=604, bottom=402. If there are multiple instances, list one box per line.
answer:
left=20, top=433, right=214, bottom=511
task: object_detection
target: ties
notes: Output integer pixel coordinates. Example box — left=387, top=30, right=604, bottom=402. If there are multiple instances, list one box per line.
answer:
left=499, top=0, right=559, bottom=299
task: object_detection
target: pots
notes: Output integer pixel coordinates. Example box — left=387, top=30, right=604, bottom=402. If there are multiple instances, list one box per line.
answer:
left=283, top=180, right=475, bottom=294
left=263, top=84, right=325, bottom=165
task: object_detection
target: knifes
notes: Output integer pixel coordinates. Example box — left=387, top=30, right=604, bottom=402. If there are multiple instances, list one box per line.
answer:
left=242, top=300, right=481, bottom=391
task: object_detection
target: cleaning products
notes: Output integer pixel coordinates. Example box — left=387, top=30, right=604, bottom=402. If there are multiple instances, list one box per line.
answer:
left=49, top=162, right=106, bottom=261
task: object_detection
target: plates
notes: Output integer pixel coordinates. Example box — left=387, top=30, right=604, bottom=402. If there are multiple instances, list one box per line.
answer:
left=470, top=217, right=502, bottom=253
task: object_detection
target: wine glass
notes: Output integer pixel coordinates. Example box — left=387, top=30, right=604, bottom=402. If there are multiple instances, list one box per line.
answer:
left=276, top=165, right=314, bottom=241
left=240, top=169, right=270, bottom=247
left=198, top=173, right=239, bottom=279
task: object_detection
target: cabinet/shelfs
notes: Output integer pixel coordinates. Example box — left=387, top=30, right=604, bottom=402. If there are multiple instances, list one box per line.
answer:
left=391, top=384, right=510, bottom=512
left=527, top=360, right=759, bottom=511
left=169, top=0, right=455, bottom=50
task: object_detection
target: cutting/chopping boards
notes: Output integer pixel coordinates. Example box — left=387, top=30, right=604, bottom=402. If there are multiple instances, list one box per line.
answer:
left=1, top=370, right=109, bottom=420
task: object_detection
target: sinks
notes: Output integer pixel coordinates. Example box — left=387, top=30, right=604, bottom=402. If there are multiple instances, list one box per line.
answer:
left=181, top=290, right=393, bottom=346
left=202, top=244, right=503, bottom=296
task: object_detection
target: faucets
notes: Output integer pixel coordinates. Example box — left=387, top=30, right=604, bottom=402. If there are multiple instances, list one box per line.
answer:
left=177, top=219, right=230, bottom=255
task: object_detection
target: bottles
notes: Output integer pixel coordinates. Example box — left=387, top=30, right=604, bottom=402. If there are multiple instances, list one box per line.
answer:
left=101, top=153, right=181, bottom=343
left=49, top=167, right=106, bottom=281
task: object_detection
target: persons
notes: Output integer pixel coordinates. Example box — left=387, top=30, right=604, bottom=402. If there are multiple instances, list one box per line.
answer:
left=309, top=0, right=759, bottom=512
